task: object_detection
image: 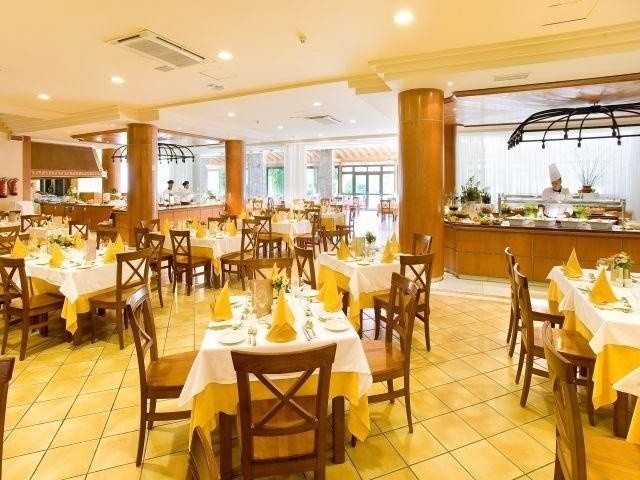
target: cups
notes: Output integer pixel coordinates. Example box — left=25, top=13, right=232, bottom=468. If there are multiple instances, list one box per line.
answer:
left=596, top=258, right=609, bottom=273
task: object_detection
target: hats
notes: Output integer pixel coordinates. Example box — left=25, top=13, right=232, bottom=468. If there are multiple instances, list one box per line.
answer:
left=549, top=164, right=561, bottom=182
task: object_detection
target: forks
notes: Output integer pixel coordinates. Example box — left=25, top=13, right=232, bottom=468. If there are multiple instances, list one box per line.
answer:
left=248, top=326, right=252, bottom=344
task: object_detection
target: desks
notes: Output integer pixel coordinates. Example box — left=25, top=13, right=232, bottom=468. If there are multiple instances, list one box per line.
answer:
left=318, top=250, right=425, bottom=340
left=1, top=243, right=146, bottom=346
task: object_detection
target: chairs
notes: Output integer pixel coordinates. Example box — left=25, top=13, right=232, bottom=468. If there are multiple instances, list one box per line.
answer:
left=546, top=342, right=640, bottom=480
left=232, top=343, right=339, bottom=479
left=412, top=232, right=433, bottom=254
left=504, top=246, right=565, bottom=357
left=88, top=247, right=153, bottom=349
left=183, top=424, right=220, bottom=480
left=373, top=253, right=433, bottom=352
left=350, top=271, right=421, bottom=445
left=512, top=263, right=598, bottom=427
left=0, top=177, right=398, bottom=296
left=1, top=356, right=17, bottom=479
left=125, top=282, right=200, bottom=469
left=144, top=234, right=165, bottom=309
left=0, top=255, right=73, bottom=360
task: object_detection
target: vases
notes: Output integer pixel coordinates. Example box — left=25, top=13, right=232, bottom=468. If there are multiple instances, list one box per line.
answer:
left=596, top=251, right=632, bottom=283
left=362, top=230, right=376, bottom=260
left=265, top=262, right=289, bottom=306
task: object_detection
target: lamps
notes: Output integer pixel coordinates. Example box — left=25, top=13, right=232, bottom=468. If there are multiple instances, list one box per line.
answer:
left=112, top=136, right=195, bottom=165
left=504, top=97, right=640, bottom=151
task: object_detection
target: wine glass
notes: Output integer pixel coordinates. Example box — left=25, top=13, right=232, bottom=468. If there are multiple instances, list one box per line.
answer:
left=257, top=290, right=269, bottom=324
left=245, top=294, right=258, bottom=323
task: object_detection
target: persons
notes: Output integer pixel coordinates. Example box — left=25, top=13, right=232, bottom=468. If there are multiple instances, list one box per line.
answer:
left=181, top=181, right=193, bottom=202
left=163, top=180, right=178, bottom=199
left=536, top=174, right=573, bottom=219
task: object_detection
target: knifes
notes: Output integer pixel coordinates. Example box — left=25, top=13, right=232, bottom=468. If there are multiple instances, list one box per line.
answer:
left=302, top=325, right=313, bottom=341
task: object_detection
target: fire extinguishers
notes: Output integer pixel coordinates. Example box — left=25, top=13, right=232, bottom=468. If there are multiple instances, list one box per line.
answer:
left=7, top=178, right=19, bottom=195
left=0, top=177, right=8, bottom=198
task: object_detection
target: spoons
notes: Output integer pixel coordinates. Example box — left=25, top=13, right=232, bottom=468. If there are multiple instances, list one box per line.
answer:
left=252, top=328, right=258, bottom=346
left=307, top=320, right=317, bottom=338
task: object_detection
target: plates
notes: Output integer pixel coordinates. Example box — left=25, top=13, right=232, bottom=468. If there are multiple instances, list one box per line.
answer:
left=26, top=244, right=128, bottom=267
left=327, top=245, right=401, bottom=265
left=216, top=330, right=247, bottom=344
left=169, top=227, right=227, bottom=237
left=577, top=283, right=592, bottom=290
left=325, top=319, right=349, bottom=330
